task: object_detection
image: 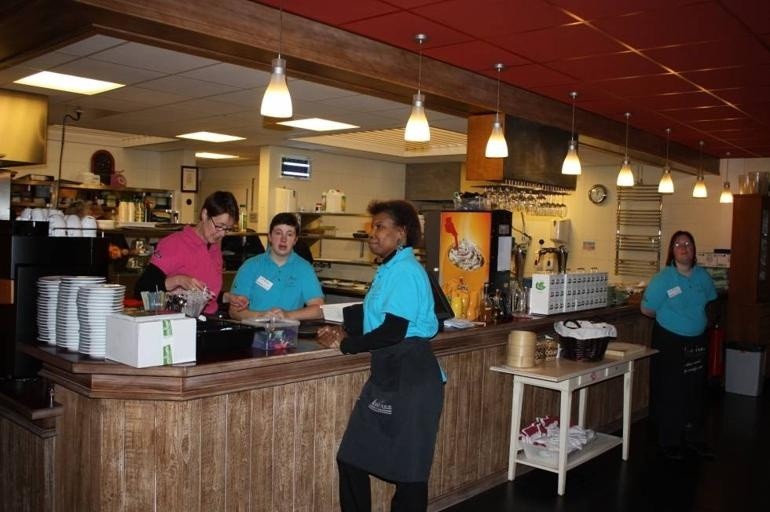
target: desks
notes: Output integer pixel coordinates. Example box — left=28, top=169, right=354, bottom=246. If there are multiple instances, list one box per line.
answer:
left=486, top=341, right=660, bottom=496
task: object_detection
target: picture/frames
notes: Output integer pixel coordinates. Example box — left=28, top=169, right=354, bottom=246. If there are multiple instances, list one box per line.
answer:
left=180, top=164, right=199, bottom=193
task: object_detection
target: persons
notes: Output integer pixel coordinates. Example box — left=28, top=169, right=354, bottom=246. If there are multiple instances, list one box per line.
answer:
left=315, top=197, right=449, bottom=512
left=133, top=190, right=251, bottom=318
left=227, top=211, right=328, bottom=323
left=639, top=230, right=721, bottom=462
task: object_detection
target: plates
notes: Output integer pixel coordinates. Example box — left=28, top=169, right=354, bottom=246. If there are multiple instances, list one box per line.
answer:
left=32, top=275, right=123, bottom=361
left=353, top=233, right=369, bottom=237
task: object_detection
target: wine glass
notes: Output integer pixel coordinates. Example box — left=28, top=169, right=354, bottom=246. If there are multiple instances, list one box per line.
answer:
left=478, top=181, right=570, bottom=218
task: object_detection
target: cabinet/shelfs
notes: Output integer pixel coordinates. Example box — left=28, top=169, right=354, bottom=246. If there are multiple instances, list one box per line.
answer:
left=717, top=192, right=768, bottom=354
left=12, top=178, right=145, bottom=214
left=291, top=212, right=435, bottom=300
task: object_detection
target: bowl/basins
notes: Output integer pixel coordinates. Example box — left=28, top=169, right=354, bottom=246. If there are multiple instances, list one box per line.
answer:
left=737, top=171, right=770, bottom=193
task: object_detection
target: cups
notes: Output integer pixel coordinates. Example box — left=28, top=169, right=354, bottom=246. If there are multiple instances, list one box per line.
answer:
left=139, top=288, right=209, bottom=316
left=22, top=207, right=97, bottom=238
left=441, top=241, right=487, bottom=296
left=610, top=278, right=645, bottom=307
left=506, top=331, right=558, bottom=368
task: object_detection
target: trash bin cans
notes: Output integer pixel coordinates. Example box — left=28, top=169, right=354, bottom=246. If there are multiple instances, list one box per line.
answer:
left=725, top=340, right=767, bottom=398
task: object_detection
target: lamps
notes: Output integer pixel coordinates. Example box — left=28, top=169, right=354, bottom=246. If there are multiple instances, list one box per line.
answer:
left=556, top=90, right=588, bottom=177
left=403, top=32, right=433, bottom=144
left=255, top=5, right=295, bottom=119
left=615, top=113, right=636, bottom=187
left=656, top=128, right=677, bottom=195
left=719, top=150, right=733, bottom=204
left=483, top=61, right=511, bottom=160
left=690, top=139, right=709, bottom=200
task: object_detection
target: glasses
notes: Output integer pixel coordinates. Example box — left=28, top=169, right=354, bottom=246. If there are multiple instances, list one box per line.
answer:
left=673, top=241, right=695, bottom=249
left=210, top=215, right=234, bottom=234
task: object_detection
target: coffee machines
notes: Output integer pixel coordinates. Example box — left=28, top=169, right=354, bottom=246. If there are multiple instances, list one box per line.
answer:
left=1, top=221, right=112, bottom=384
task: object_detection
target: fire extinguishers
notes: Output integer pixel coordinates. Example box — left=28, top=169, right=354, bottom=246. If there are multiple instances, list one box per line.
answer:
left=706, top=321, right=725, bottom=377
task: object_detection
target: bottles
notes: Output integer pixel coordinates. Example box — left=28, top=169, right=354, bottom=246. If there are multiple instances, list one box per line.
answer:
left=315, top=192, right=326, bottom=212
left=118, top=191, right=154, bottom=225
left=451, top=278, right=529, bottom=321
left=238, top=202, right=248, bottom=232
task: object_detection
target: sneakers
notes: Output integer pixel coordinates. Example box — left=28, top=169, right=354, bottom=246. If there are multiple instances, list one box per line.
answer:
left=655, top=439, right=718, bottom=461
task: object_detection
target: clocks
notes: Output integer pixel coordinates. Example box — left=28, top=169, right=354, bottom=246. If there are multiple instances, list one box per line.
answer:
left=587, top=183, right=608, bottom=205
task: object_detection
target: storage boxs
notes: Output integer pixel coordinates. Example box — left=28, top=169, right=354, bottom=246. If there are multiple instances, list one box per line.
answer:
left=102, top=306, right=197, bottom=371
